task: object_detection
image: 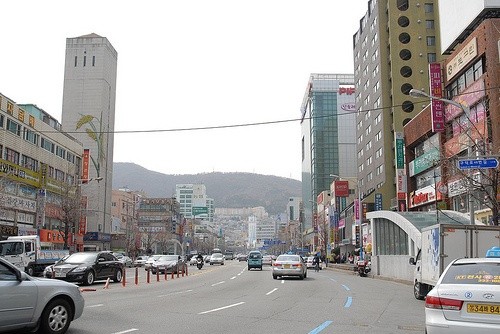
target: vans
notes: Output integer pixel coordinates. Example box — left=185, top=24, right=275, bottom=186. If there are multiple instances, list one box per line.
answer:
left=248, top=251, right=263, bottom=271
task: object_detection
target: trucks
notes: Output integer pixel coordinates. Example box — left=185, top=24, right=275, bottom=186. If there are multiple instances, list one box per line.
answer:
left=409, top=224, right=500, bottom=301
left=1, top=235, right=70, bottom=277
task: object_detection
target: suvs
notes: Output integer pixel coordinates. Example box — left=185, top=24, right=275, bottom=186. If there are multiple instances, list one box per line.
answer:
left=210, top=253, right=224, bottom=265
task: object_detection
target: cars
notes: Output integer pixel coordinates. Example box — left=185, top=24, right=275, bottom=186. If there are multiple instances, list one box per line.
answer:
left=206, top=256, right=212, bottom=263
left=134, top=256, right=149, bottom=266
left=0, top=258, right=84, bottom=334
left=145, top=255, right=165, bottom=271
left=116, top=255, right=132, bottom=267
left=304, top=257, right=317, bottom=269
left=44, top=255, right=71, bottom=277
left=151, top=255, right=187, bottom=274
left=261, top=256, right=272, bottom=266
left=224, top=249, right=247, bottom=262
left=424, top=247, right=500, bottom=334
left=272, top=255, right=308, bottom=280
left=189, top=255, right=204, bottom=265
left=46, top=251, right=125, bottom=285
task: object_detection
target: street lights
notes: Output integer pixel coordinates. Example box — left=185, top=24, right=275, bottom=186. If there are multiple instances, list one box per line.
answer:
left=411, top=89, right=474, bottom=225
left=330, top=174, right=363, bottom=260
left=74, top=177, right=103, bottom=253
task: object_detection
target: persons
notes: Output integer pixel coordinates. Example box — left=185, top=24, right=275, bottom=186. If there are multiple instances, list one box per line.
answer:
left=312, top=250, right=357, bottom=272
left=196, top=252, right=204, bottom=265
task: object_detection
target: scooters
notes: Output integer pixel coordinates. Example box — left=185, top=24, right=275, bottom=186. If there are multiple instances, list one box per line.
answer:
left=356, top=260, right=367, bottom=277
left=196, top=258, right=203, bottom=269
left=331, top=251, right=354, bottom=264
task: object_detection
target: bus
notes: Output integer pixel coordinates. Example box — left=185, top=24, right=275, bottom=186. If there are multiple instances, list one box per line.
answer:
left=210, top=249, right=222, bottom=255
